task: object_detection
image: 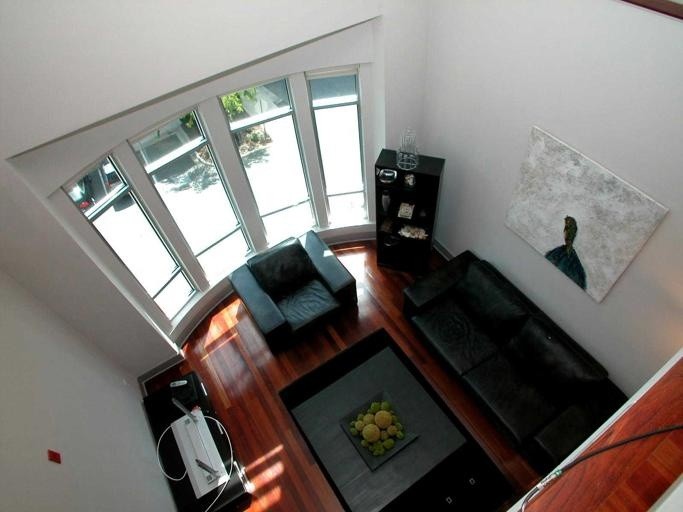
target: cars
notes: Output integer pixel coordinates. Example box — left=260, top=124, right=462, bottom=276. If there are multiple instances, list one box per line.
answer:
left=100, top=162, right=129, bottom=200
left=65, top=173, right=95, bottom=213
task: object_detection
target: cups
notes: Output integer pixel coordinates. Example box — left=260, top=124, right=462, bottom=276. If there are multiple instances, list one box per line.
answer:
left=405, top=175, right=416, bottom=186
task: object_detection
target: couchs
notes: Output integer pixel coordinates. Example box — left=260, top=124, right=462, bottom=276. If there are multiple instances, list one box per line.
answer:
left=402, top=250, right=609, bottom=454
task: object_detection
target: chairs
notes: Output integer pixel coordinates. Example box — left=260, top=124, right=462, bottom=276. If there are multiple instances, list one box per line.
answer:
left=226, top=231, right=358, bottom=356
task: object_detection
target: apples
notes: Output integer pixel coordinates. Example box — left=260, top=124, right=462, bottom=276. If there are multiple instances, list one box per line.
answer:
left=349, top=399, right=405, bottom=457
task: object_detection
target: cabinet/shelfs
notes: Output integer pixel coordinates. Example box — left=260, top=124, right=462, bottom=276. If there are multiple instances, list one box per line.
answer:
left=142, top=371, right=254, bottom=512
left=373, top=150, right=444, bottom=275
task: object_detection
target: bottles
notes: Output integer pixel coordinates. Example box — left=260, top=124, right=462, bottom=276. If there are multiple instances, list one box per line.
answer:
left=381, top=191, right=391, bottom=213
